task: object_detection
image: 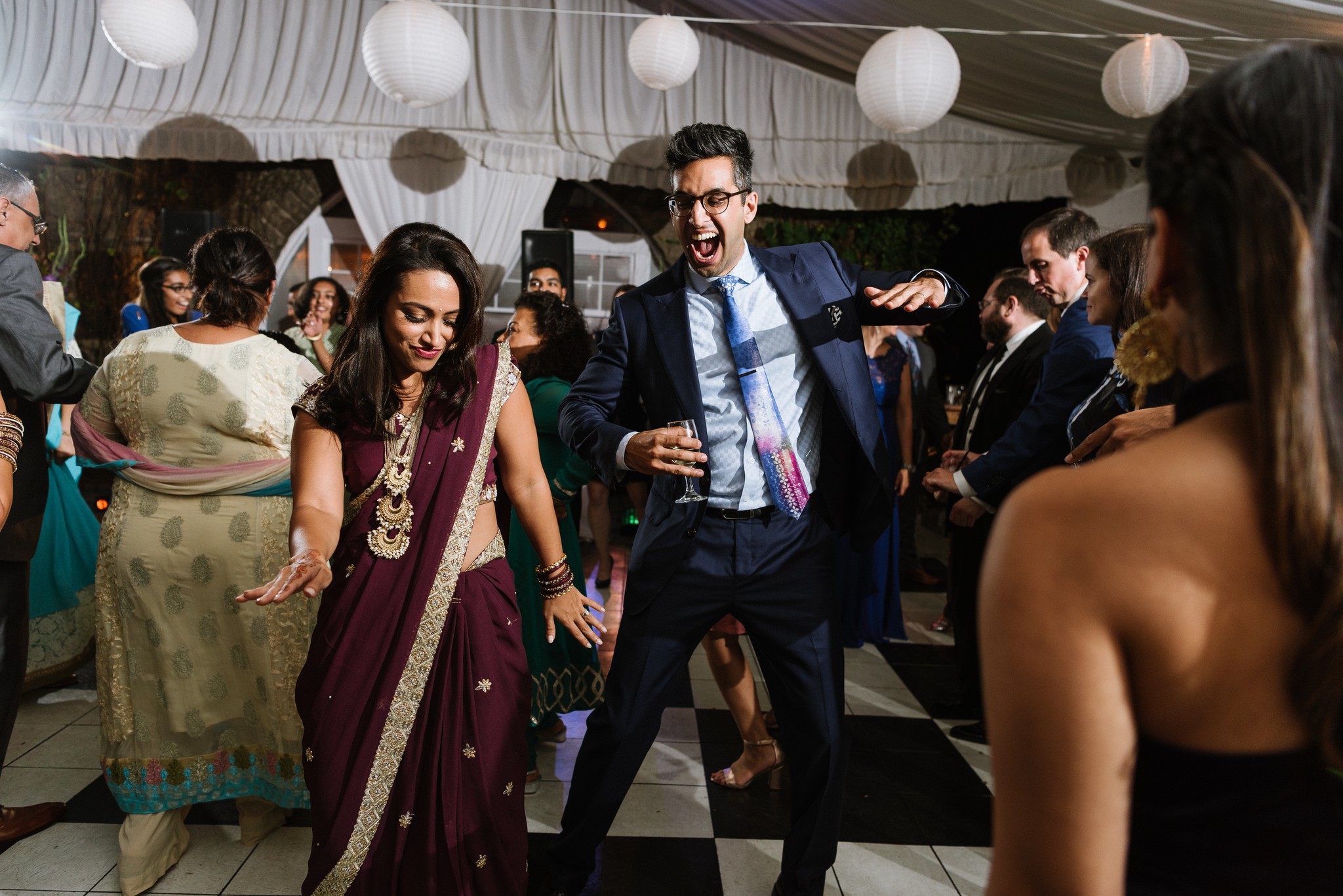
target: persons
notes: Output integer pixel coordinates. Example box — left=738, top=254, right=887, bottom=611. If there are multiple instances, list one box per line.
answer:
left=1062, top=405, right=1176, bottom=466
left=923, top=268, right=1054, bottom=745
left=527, top=123, right=977, bottom=894
left=71, top=228, right=325, bottom=895
left=27, top=282, right=103, bottom=700
left=0, top=392, right=13, bottom=532
left=0, top=162, right=107, bottom=838
left=897, top=323, right=947, bottom=588
left=233, top=221, right=609, bottom=896
left=1068, top=224, right=1154, bottom=468
left=496, top=291, right=603, bottom=794
left=279, top=282, right=305, bottom=334
left=587, top=284, right=648, bottom=588
left=840, top=325, right=914, bottom=649
left=980, top=33, right=1343, bottom=896
left=284, top=277, right=350, bottom=375
left=522, top=260, right=567, bottom=301
left=920, top=205, right=1115, bottom=527
left=122, top=255, right=206, bottom=335
left=700, top=611, right=788, bottom=792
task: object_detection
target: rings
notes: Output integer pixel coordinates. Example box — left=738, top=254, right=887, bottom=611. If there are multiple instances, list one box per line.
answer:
left=913, top=292, right=925, bottom=303
left=581, top=608, right=590, bottom=618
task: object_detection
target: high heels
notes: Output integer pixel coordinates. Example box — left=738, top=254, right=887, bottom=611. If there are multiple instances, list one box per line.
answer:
left=760, top=712, right=779, bottom=729
left=525, top=769, right=542, bottom=794
left=710, top=736, right=784, bottom=790
left=538, top=715, right=567, bottom=744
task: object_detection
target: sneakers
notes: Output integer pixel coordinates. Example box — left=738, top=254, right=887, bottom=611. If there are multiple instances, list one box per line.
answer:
left=932, top=616, right=953, bottom=632
left=950, top=721, right=990, bottom=746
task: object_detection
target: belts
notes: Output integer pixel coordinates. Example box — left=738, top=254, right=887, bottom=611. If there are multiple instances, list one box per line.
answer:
left=706, top=493, right=810, bottom=519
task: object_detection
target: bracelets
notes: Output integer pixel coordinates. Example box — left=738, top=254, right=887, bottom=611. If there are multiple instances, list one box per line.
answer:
left=0, top=412, right=25, bottom=474
left=534, top=553, right=574, bottom=599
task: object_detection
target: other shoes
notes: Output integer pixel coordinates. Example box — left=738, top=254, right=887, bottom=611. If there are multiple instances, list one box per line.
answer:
left=595, top=554, right=614, bottom=588
left=546, top=879, right=604, bottom=896
left=0, top=801, right=68, bottom=843
left=897, top=564, right=947, bottom=592
left=43, top=673, right=77, bottom=688
left=771, top=882, right=782, bottom=896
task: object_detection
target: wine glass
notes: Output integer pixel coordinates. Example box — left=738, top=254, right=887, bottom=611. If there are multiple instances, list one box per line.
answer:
left=667, top=419, right=708, bottom=503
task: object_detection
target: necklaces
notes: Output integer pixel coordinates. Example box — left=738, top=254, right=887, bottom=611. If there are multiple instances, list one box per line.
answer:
left=367, top=372, right=438, bottom=560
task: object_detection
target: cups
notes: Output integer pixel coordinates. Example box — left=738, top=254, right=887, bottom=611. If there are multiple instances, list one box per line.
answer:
left=942, top=458, right=967, bottom=473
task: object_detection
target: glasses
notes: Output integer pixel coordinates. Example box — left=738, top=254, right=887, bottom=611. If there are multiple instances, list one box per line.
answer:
left=162, top=285, right=193, bottom=293
left=978, top=297, right=1007, bottom=310
left=663, top=188, right=752, bottom=215
left=10, top=201, right=49, bottom=235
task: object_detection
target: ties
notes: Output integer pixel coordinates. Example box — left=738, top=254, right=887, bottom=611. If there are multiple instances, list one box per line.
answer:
left=958, top=346, right=1007, bottom=451
left=905, top=339, right=920, bottom=392
left=714, top=275, right=810, bottom=522
